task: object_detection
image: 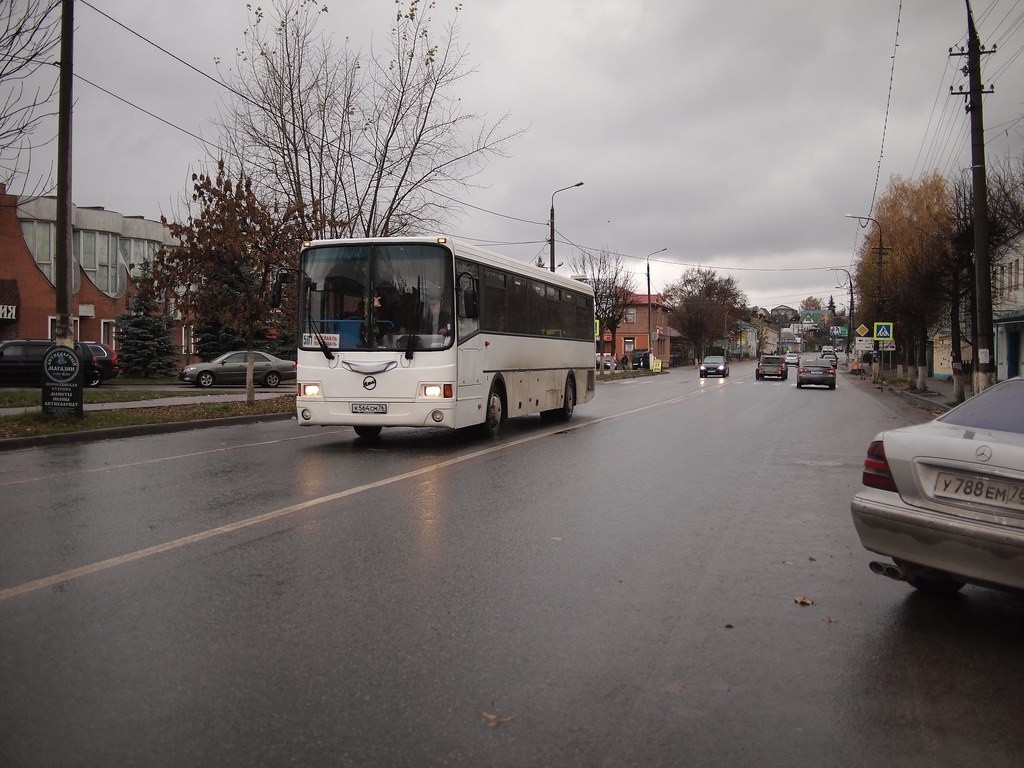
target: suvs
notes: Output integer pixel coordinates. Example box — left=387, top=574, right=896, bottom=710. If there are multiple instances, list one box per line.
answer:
left=0, top=338, right=99, bottom=389
left=756, top=356, right=788, bottom=381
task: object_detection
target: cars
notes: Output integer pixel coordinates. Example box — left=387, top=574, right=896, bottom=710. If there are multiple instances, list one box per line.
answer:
left=699, top=356, right=729, bottom=379
left=795, top=358, right=836, bottom=391
left=79, top=341, right=120, bottom=388
left=850, top=375, right=1023, bottom=600
left=625, top=348, right=652, bottom=369
left=784, top=354, right=801, bottom=368
left=178, top=351, right=297, bottom=389
left=820, top=346, right=853, bottom=370
left=595, top=359, right=617, bottom=370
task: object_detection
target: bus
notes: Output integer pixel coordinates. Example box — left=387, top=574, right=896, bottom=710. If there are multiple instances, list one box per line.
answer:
left=269, top=235, right=596, bottom=440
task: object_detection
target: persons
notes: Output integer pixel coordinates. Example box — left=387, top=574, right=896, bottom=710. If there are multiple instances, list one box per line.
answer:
left=422, top=297, right=449, bottom=336
left=622, top=355, right=628, bottom=370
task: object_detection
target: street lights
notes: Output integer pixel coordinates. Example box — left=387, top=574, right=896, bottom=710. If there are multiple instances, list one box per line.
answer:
left=844, top=214, right=883, bottom=384
left=647, top=247, right=668, bottom=351
left=549, top=182, right=584, bottom=273
left=827, top=267, right=854, bottom=313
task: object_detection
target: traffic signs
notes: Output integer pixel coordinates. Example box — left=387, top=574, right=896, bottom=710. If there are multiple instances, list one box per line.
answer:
left=855, top=337, right=874, bottom=344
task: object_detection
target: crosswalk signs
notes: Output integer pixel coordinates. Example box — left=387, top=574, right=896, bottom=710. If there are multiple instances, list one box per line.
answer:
left=877, top=324, right=890, bottom=338
